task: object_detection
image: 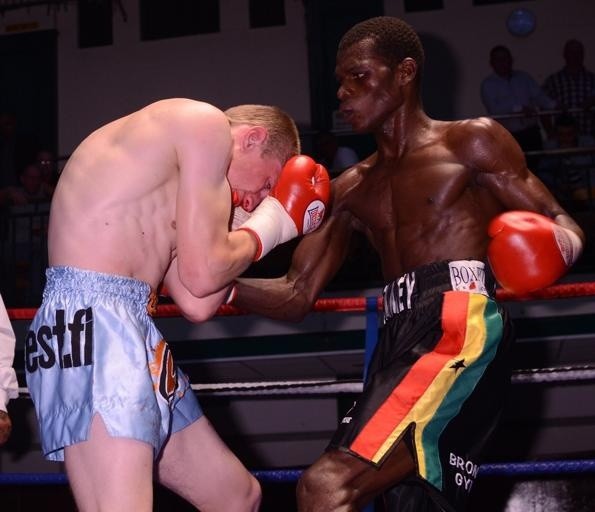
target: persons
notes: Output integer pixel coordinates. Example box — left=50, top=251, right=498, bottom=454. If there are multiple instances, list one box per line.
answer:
left=0, top=161, right=54, bottom=307
left=22, top=97, right=332, bottom=510
left=537, top=39, right=595, bottom=140
left=218, top=16, right=590, bottom=512
left=33, top=148, right=63, bottom=201
left=534, top=112, right=595, bottom=210
left=0, top=291, right=21, bottom=446
left=309, top=128, right=360, bottom=179
left=480, top=45, right=568, bottom=154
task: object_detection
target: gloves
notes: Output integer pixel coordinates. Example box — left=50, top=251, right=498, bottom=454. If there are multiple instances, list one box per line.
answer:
left=486, top=211, right=582, bottom=294
left=236, top=156, right=331, bottom=263
